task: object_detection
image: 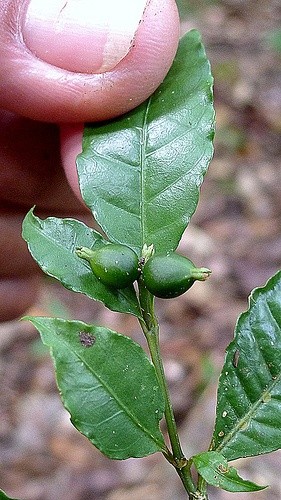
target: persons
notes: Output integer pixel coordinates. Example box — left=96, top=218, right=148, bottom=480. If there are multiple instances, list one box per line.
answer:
left=0, top=1, right=181, bottom=324
